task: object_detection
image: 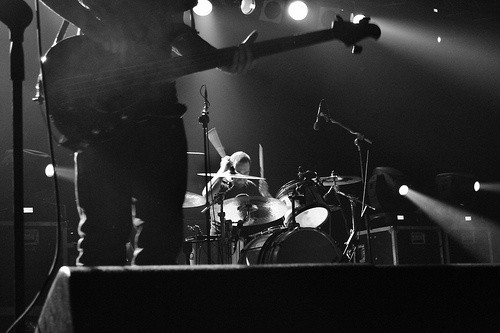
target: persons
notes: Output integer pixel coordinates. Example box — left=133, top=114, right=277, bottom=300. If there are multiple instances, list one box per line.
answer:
left=202, top=152, right=273, bottom=240
left=40, top=0, right=258, bottom=266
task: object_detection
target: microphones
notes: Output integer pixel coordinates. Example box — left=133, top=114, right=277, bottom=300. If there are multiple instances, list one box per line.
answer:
left=314, top=101, right=326, bottom=131
left=198, top=91, right=210, bottom=123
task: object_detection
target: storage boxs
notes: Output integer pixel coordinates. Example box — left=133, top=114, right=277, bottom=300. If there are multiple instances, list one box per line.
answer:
left=352, top=225, right=494, bottom=264
left=0, top=222, right=66, bottom=317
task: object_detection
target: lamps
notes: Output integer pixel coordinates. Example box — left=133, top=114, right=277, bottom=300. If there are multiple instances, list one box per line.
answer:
left=27, top=154, right=55, bottom=177
left=367, top=168, right=408, bottom=211
left=439, top=173, right=481, bottom=197
left=193, top=0, right=365, bottom=28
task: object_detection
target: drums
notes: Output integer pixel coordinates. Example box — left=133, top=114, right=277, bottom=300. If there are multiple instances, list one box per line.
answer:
left=277, top=181, right=329, bottom=228
left=237, top=228, right=343, bottom=264
left=186, top=236, right=246, bottom=265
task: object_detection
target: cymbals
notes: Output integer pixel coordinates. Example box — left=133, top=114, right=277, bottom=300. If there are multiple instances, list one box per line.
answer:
left=183, top=192, right=207, bottom=209
left=313, top=175, right=362, bottom=186
left=197, top=173, right=267, bottom=181
left=326, top=204, right=341, bottom=212
left=211, top=195, right=289, bottom=226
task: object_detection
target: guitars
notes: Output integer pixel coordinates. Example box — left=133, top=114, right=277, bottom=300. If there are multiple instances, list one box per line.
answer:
left=36, top=12, right=382, bottom=152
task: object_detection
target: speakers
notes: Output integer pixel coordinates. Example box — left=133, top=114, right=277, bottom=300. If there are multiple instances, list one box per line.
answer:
left=35, top=264, right=500, bottom=333
